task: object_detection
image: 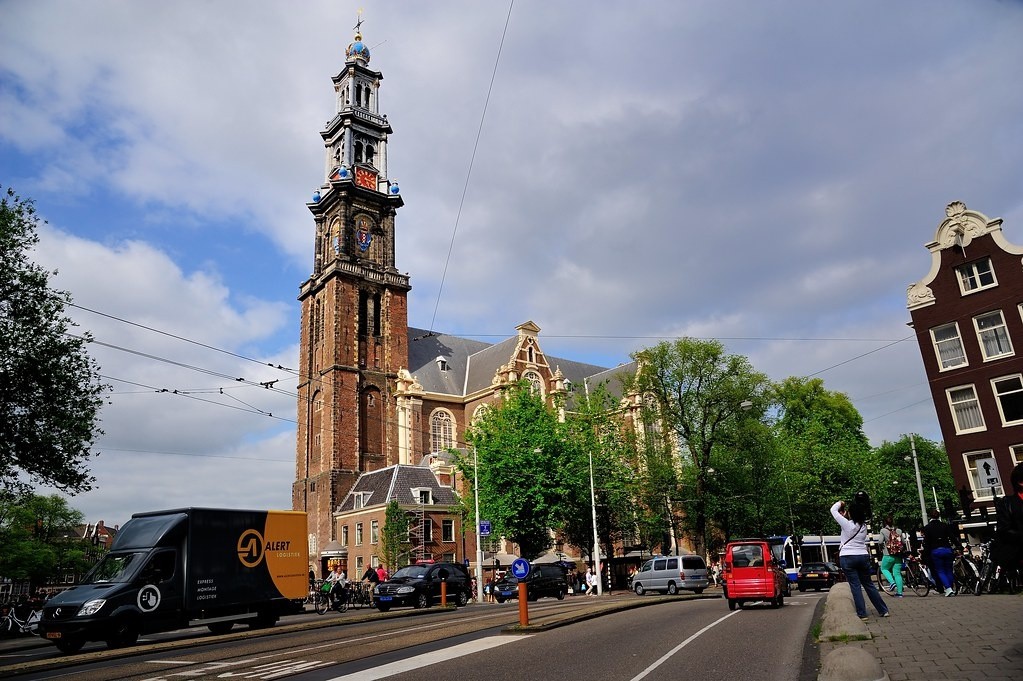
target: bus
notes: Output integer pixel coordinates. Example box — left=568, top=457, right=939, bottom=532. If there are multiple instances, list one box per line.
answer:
left=736, top=531, right=924, bottom=590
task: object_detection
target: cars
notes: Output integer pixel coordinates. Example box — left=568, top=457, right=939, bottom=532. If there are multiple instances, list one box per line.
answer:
left=797, top=562, right=837, bottom=592
left=720, top=550, right=788, bottom=599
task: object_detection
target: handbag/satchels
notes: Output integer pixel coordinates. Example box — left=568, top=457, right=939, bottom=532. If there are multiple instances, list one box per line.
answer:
left=321, top=581, right=331, bottom=592
left=835, top=552, right=841, bottom=568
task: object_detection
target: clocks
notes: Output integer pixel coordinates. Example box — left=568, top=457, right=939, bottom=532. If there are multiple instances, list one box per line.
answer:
left=355, top=166, right=377, bottom=190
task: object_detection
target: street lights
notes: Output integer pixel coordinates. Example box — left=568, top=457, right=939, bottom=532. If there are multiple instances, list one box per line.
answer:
left=553, top=538, right=565, bottom=561
left=489, top=550, right=497, bottom=580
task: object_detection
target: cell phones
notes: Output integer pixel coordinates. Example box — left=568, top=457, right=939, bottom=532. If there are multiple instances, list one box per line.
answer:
left=841, top=505, right=845, bottom=513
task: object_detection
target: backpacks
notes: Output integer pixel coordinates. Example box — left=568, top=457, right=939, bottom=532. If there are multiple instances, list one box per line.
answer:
left=885, top=526, right=905, bottom=555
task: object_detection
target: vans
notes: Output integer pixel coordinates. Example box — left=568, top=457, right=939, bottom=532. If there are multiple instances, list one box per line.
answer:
left=725, top=541, right=786, bottom=610
left=632, top=555, right=710, bottom=595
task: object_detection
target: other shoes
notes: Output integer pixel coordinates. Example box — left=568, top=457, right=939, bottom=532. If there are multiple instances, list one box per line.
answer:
left=894, top=594, right=902, bottom=597
left=861, top=617, right=868, bottom=621
left=884, top=612, right=889, bottom=617
left=945, top=587, right=956, bottom=596
left=889, top=583, right=897, bottom=591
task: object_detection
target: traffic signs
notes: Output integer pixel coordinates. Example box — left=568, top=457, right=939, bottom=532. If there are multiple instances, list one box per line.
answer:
left=974, top=457, right=1002, bottom=489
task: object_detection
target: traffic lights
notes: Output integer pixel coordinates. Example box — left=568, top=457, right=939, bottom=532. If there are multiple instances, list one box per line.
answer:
left=980, top=506, right=989, bottom=521
left=959, top=489, right=975, bottom=510
left=1011, top=462, right=1023, bottom=515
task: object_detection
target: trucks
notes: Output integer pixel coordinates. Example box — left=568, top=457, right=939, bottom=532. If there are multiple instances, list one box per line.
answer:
left=38, top=507, right=310, bottom=652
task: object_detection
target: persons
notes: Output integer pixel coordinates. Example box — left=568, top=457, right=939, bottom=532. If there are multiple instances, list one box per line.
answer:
left=361, top=563, right=379, bottom=605
left=630, top=567, right=638, bottom=577
left=486, top=583, right=491, bottom=602
left=923, top=510, right=968, bottom=597
left=879, top=516, right=904, bottom=597
left=569, top=568, right=597, bottom=596
left=734, top=547, right=762, bottom=566
left=830, top=500, right=890, bottom=620
left=376, top=564, right=388, bottom=583
left=326, top=564, right=345, bottom=603
left=711, top=561, right=720, bottom=588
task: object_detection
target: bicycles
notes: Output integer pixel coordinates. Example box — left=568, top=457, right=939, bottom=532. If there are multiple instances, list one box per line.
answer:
left=626, top=576, right=631, bottom=592
left=303, top=579, right=377, bottom=615
left=877, top=539, right=1020, bottom=597
left=0, top=594, right=44, bottom=640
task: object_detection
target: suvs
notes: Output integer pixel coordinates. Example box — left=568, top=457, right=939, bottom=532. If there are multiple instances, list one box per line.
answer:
left=373, top=560, right=473, bottom=612
left=493, top=562, right=568, bottom=604
